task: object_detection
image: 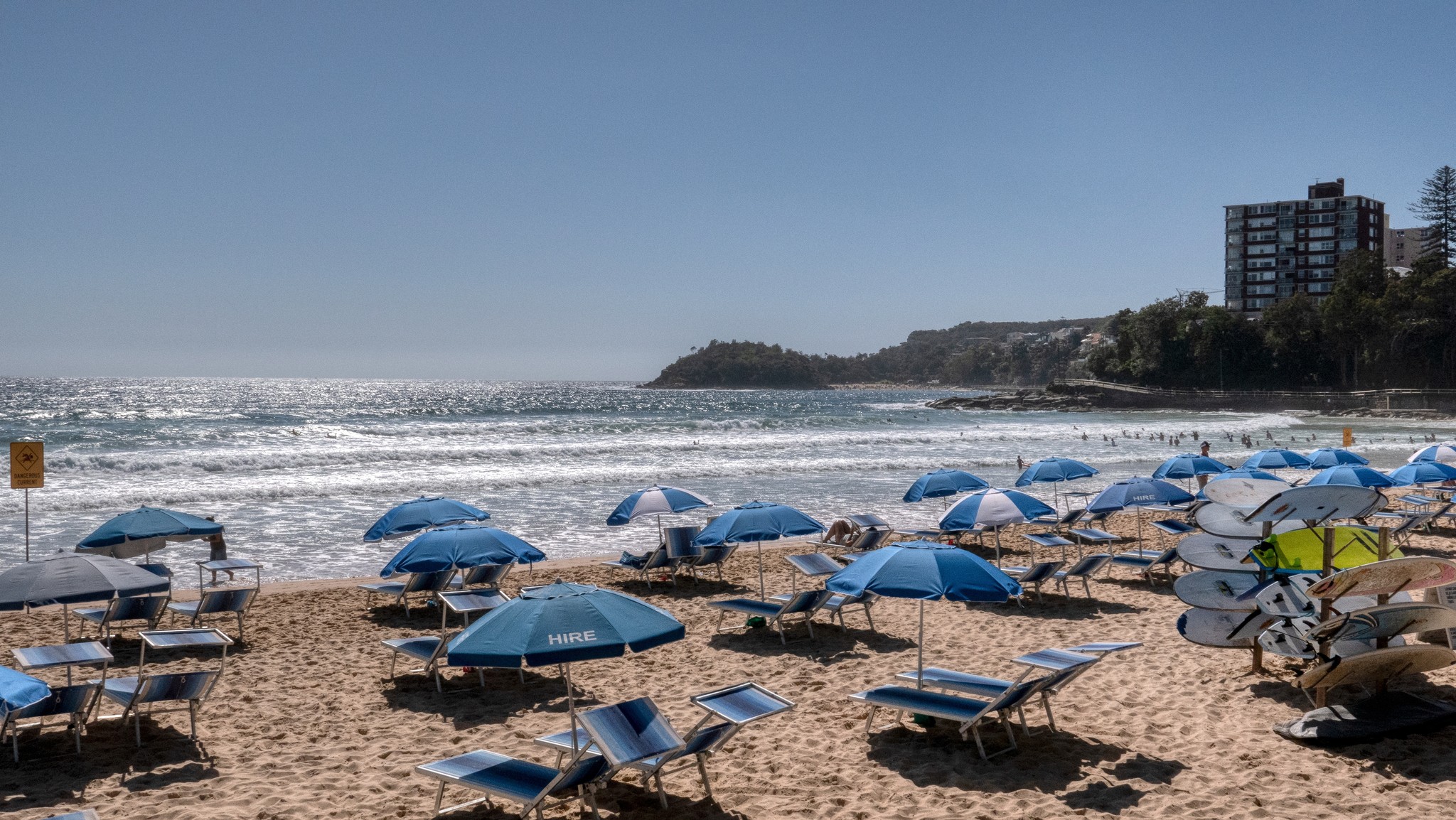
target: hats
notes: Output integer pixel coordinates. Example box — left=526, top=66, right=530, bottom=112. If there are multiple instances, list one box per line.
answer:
left=1200, top=441, right=1212, bottom=448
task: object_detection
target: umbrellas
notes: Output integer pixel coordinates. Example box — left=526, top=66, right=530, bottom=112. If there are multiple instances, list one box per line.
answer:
left=606, top=482, right=714, bottom=580
left=1239, top=447, right=1314, bottom=476
left=1381, top=459, right=1456, bottom=496
left=902, top=467, right=991, bottom=541
left=1406, top=442, right=1456, bottom=468
left=1014, top=455, right=1099, bottom=521
left=1086, top=475, right=1196, bottom=561
left=1294, top=446, right=1370, bottom=474
left=1300, top=462, right=1398, bottom=527
left=74, top=504, right=226, bottom=632
left=1151, top=453, right=1233, bottom=506
left=825, top=536, right=1025, bottom=688
left=1193, top=465, right=1297, bottom=502
left=362, top=495, right=491, bottom=543
left=443, top=578, right=688, bottom=820
left=935, top=485, right=1058, bottom=569
left=690, top=499, right=829, bottom=603
left=379, top=521, right=549, bottom=670
left=0, top=549, right=172, bottom=731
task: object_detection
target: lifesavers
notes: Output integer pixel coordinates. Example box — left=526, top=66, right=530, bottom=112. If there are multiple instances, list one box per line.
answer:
left=1021, top=462, right=1032, bottom=467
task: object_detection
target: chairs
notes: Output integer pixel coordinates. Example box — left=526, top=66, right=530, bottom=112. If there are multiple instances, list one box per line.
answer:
left=412, top=695, right=689, bottom=820
left=514, top=582, right=579, bottom=688
left=706, top=550, right=848, bottom=645
left=422, top=561, right=516, bottom=601
left=806, top=513, right=895, bottom=560
left=0, top=641, right=115, bottom=763
left=356, top=563, right=459, bottom=618
left=532, top=681, right=799, bottom=810
left=896, top=640, right=1143, bottom=740
left=70, top=562, right=174, bottom=638
left=769, top=550, right=883, bottom=637
left=654, top=515, right=741, bottom=584
left=890, top=485, right=1456, bottom=612
left=380, top=589, right=512, bottom=694
left=845, top=646, right=1104, bottom=764
left=86, top=627, right=235, bottom=754
left=599, top=524, right=704, bottom=591
left=160, top=558, right=264, bottom=638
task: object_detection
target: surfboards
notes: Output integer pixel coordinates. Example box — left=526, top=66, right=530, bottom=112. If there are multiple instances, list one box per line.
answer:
left=1304, top=555, right=1455, bottom=599
left=1202, top=477, right=1295, bottom=510
left=1255, top=567, right=1414, bottom=619
left=1175, top=532, right=1263, bottom=574
left=1301, top=600, right=1456, bottom=642
left=1194, top=501, right=1328, bottom=540
left=1176, top=607, right=1286, bottom=650
left=1238, top=525, right=1407, bottom=575
left=1243, top=483, right=1390, bottom=523
left=1257, top=611, right=1408, bottom=660
left=1292, top=646, right=1456, bottom=690
left=1172, top=569, right=1260, bottom=614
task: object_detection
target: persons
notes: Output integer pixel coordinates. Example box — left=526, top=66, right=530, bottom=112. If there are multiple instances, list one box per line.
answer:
left=201, top=515, right=234, bottom=583
left=884, top=406, right=1456, bottom=447
left=1194, top=440, right=1212, bottom=490
left=816, top=519, right=878, bottom=547
left=1017, top=455, right=1024, bottom=470
